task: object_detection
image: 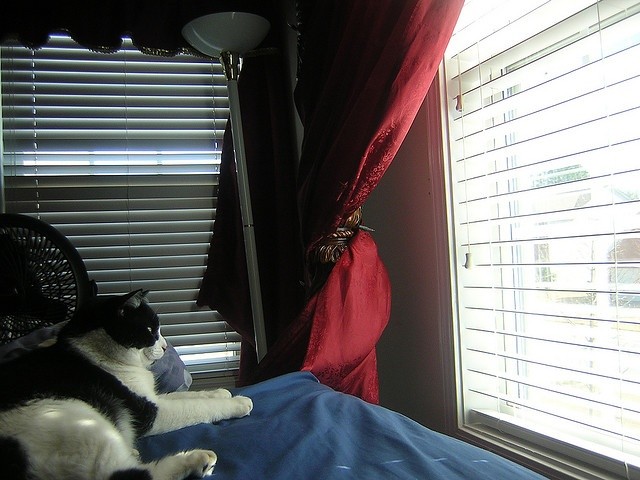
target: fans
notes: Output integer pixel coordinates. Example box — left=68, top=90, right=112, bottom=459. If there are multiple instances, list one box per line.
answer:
left=0, top=212, right=97, bottom=350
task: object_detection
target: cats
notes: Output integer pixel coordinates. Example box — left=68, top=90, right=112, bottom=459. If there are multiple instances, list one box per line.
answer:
left=1, top=289, right=253, bottom=480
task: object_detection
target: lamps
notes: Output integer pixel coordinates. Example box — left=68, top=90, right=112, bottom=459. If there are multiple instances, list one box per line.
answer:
left=182, top=10, right=271, bottom=365
